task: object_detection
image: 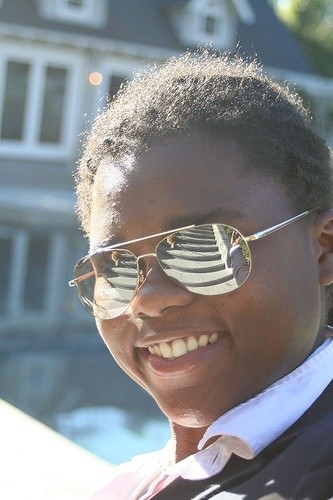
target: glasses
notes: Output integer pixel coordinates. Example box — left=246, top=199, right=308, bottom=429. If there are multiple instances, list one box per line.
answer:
left=68, top=206, right=322, bottom=321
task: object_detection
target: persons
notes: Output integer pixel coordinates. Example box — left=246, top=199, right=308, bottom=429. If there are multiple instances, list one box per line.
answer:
left=76, top=39, right=333, bottom=500
